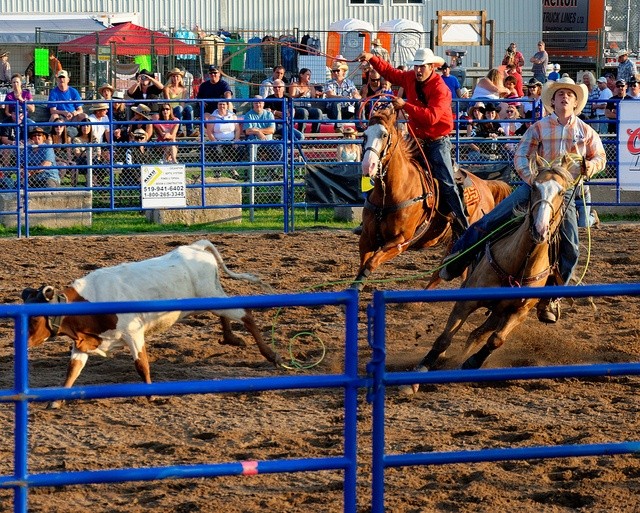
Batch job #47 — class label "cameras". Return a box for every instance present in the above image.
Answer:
[141,76,146,80]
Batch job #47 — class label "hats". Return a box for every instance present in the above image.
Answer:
[405,48,445,65]
[616,77,627,83]
[437,61,450,69]
[98,82,115,93]
[130,128,145,136]
[58,69,69,78]
[519,110,532,118]
[89,98,109,110]
[0,47,11,58]
[167,67,185,76]
[479,102,501,114]
[208,64,219,70]
[616,48,627,58]
[33,127,48,134]
[136,68,154,79]
[329,61,348,70]
[596,76,607,83]
[553,63,561,69]
[468,101,486,114]
[460,87,468,97]
[371,38,382,45]
[525,77,543,87]
[131,103,152,119]
[627,76,640,84]
[541,76,589,115]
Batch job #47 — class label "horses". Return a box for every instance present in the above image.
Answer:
[397,151,575,398]
[350,108,512,312]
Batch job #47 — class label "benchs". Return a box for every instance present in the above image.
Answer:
[450,129,468,157]
[149,135,282,158]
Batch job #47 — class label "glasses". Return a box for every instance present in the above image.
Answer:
[616,84,625,87]
[628,83,636,86]
[134,136,144,139]
[208,71,219,74]
[162,107,171,110]
[505,108,514,113]
[331,69,341,72]
[370,76,381,82]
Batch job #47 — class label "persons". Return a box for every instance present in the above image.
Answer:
[592,77,613,133]
[0,105,24,146]
[468,103,503,160]
[25,61,35,86]
[605,73,618,96]
[336,127,361,163]
[0,50,12,87]
[23,127,60,189]
[127,69,163,111]
[242,95,284,180]
[419,76,607,323]
[163,67,199,137]
[514,109,534,137]
[548,64,561,80]
[509,43,524,75]
[467,101,486,151]
[615,49,637,82]
[324,61,362,132]
[154,104,180,163]
[369,38,391,65]
[206,97,241,180]
[504,76,519,106]
[627,77,639,99]
[98,83,115,117]
[73,119,105,187]
[471,69,512,107]
[50,50,63,82]
[104,92,129,142]
[397,65,405,72]
[521,78,542,113]
[534,107,541,119]
[360,69,383,116]
[122,129,151,186]
[331,55,346,78]
[48,70,89,122]
[581,72,600,133]
[530,40,548,83]
[121,104,152,142]
[506,64,525,97]
[440,62,462,98]
[378,79,395,99]
[5,73,35,117]
[562,73,569,78]
[453,87,470,119]
[503,47,518,71]
[86,103,110,160]
[500,104,524,152]
[354,48,470,238]
[264,79,304,141]
[289,68,323,139]
[605,78,633,133]
[48,118,78,187]
[197,65,234,113]
[259,66,285,98]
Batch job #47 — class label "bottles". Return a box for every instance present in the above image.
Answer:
[126,147,133,165]
[490,137,497,160]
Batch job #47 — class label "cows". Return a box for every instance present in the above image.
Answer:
[20,239,283,409]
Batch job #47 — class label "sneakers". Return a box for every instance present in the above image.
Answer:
[441,254,457,281]
[590,208,601,229]
[537,281,558,322]
